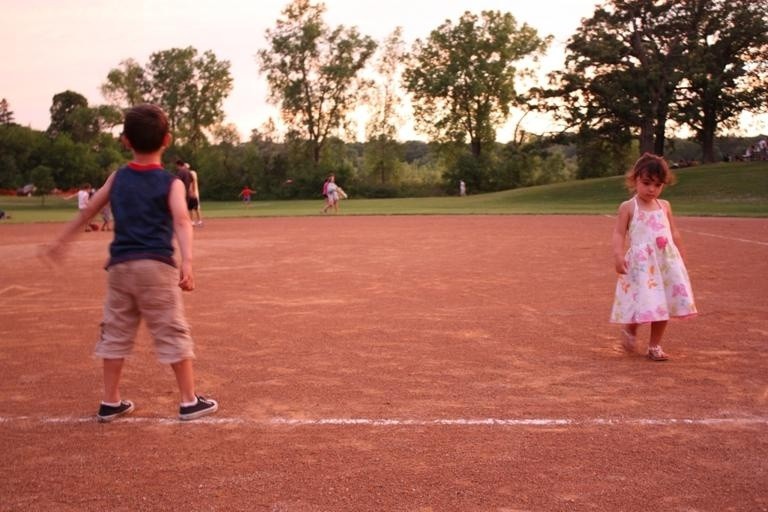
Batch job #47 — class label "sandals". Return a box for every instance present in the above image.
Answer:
[622,329,669,361]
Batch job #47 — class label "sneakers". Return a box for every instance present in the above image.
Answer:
[97,400,135,421]
[178,393,218,420]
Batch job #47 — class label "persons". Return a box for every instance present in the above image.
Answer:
[612,153,697,361]
[459,179,466,198]
[672,159,696,167]
[320,173,343,215]
[39,105,218,423]
[238,186,257,205]
[723,138,768,162]
[77,161,201,233]
